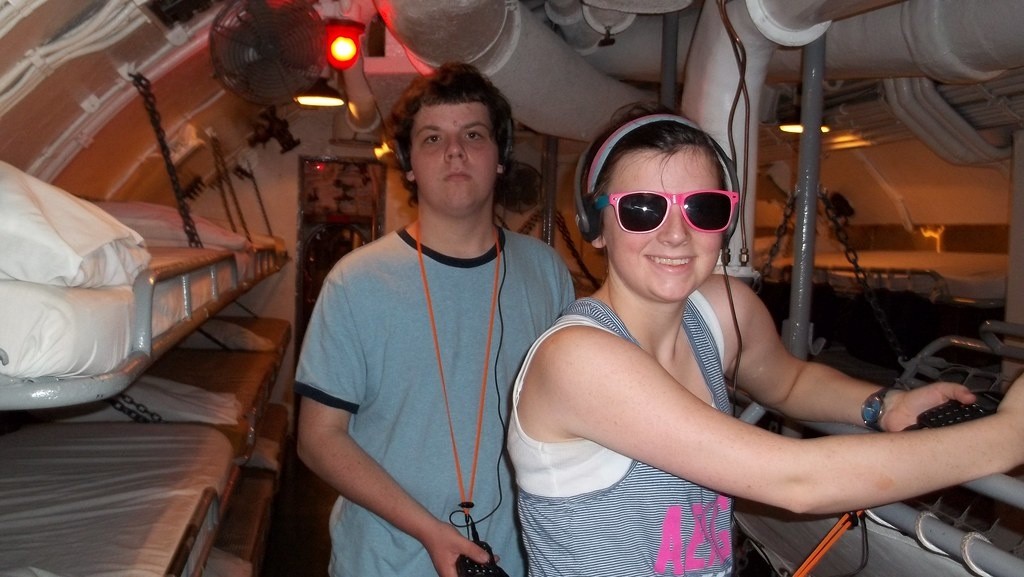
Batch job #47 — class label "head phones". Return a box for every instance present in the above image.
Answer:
[390,117,515,173]
[573,134,739,248]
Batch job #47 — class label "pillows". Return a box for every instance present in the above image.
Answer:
[83,199,246,250]
[243,434,282,472]
[182,325,273,350]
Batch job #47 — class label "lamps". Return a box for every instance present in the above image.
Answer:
[296,76,343,106]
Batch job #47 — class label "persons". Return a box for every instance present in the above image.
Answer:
[506,102,1024,577]
[291,62,575,577]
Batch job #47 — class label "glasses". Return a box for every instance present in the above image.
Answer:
[596,189,740,235]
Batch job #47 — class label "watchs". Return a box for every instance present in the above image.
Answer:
[861,386,892,432]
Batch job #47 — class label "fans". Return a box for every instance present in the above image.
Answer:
[204,0,328,109]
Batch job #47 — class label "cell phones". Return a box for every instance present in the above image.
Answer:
[916,392,1008,431]
[458,540,499,577]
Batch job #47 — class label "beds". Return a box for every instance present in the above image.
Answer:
[0,233,286,410]
[0,317,293,577]
[201,401,292,577]
